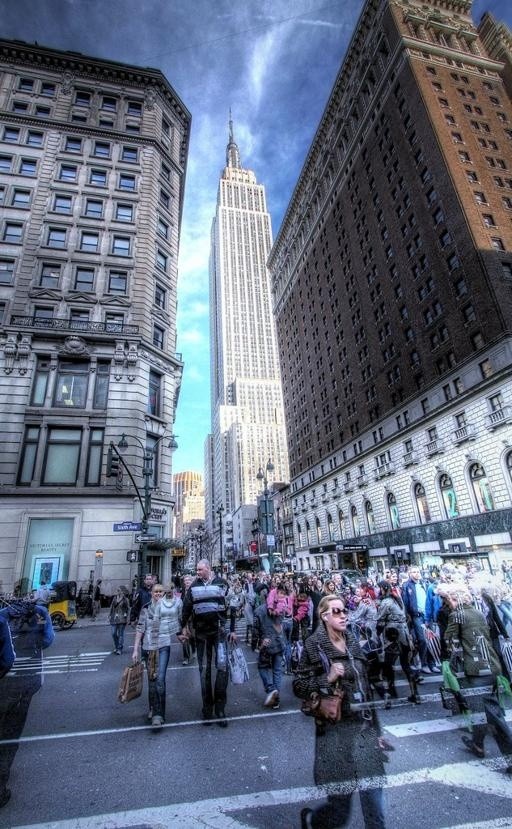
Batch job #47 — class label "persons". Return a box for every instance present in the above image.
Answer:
[0,560,512,829]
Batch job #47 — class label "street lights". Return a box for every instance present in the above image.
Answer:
[217,503,225,580]
[118,432,180,619]
[196,523,206,559]
[256,456,275,575]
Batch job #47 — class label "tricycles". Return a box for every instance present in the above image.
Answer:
[9,579,77,634]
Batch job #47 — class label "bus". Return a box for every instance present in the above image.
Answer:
[235,553,282,572]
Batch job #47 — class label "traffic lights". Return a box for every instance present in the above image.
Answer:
[106,451,118,477]
[128,549,141,561]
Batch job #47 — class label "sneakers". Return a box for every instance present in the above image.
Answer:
[152,716,163,732]
[262,689,282,710]
[408,695,421,704]
[462,736,484,758]
[148,710,152,718]
[432,667,441,672]
[113,647,121,655]
[422,666,432,674]
[182,653,196,665]
[393,665,422,671]
[385,693,392,709]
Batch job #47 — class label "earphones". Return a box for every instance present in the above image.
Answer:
[322,616,329,622]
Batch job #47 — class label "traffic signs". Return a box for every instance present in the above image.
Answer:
[114,521,142,531]
[135,534,156,542]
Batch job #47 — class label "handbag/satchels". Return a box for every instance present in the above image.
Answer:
[301,688,344,723]
[116,661,144,705]
[258,638,272,650]
[228,647,249,685]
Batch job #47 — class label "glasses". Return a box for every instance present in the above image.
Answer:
[322,607,349,615]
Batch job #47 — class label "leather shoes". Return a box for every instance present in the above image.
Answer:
[301,808,313,829]
[203,711,213,726]
[215,707,227,728]
